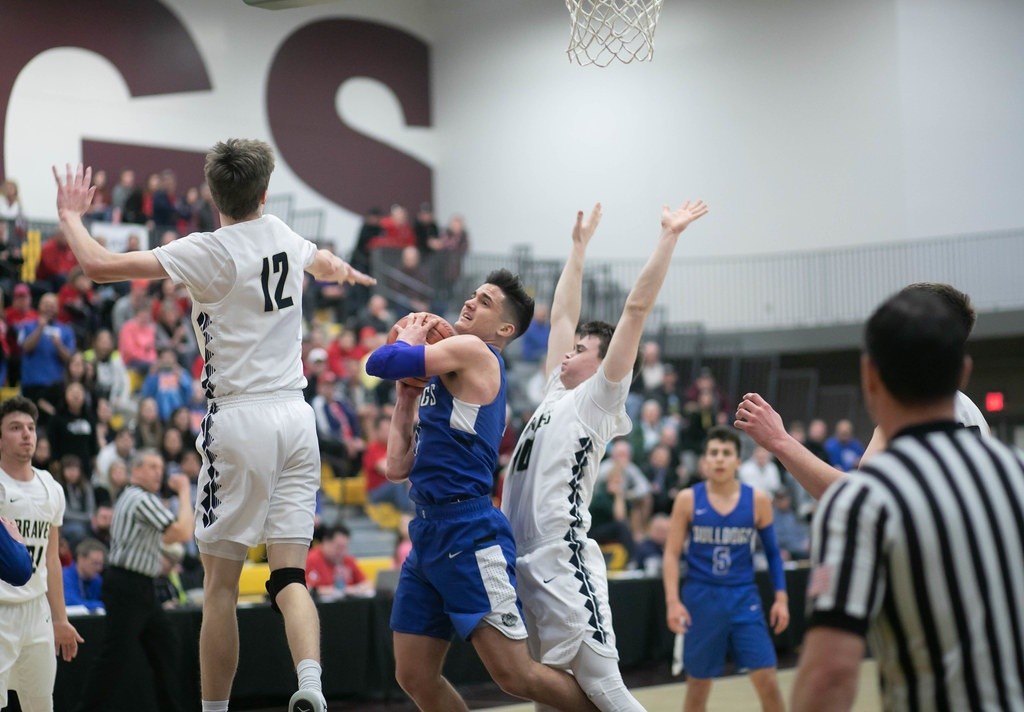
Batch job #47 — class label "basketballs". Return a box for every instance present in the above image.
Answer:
[385,310,458,387]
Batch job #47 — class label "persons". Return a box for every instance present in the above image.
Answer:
[662,428,789,712]
[501,200,710,712]
[366,267,599,712]
[51,139,375,712]
[733,284,1024,712]
[0,168,734,712]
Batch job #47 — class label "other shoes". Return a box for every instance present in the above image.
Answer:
[289,689,327,712]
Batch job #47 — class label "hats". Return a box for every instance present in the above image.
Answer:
[14,283,30,296]
[131,279,150,289]
[308,347,328,363]
[317,371,336,382]
